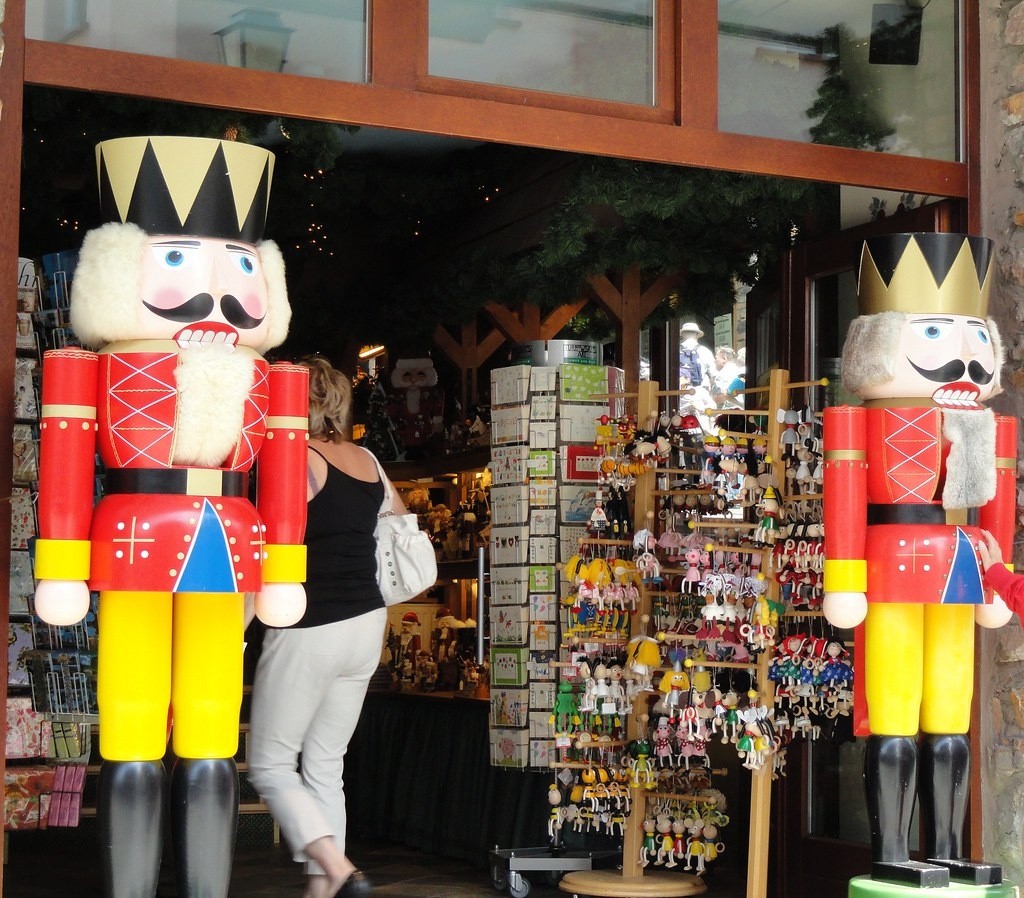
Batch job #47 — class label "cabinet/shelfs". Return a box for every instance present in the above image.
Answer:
[371,445,492,698]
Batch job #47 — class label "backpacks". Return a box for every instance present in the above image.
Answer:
[680,343,703,389]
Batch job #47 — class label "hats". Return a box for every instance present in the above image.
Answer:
[680,322,704,338]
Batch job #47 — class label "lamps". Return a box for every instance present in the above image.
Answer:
[208,7,300,75]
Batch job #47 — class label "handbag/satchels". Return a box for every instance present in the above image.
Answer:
[362,444,439,607]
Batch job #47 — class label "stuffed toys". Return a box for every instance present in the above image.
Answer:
[547,406,855,878]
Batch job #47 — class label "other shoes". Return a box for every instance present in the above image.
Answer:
[333,869,372,898]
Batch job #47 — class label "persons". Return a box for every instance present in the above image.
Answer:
[31,136,307,898]
[821,231,1018,884]
[244,355,413,898]
